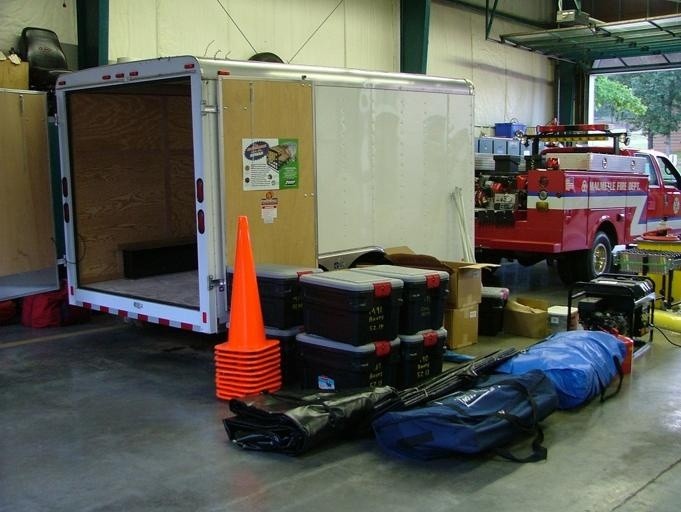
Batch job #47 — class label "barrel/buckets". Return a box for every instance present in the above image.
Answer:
[547,305,579,337]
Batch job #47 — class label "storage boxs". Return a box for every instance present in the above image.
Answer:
[344,264,451,337]
[385,247,501,308]
[225,321,301,388]
[295,331,401,391]
[548,306,579,331]
[301,269,403,347]
[474,123,544,171]
[445,304,479,349]
[504,297,548,337]
[397,328,448,389]
[226,260,323,331]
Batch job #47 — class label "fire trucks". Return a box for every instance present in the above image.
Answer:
[473,117,680,283]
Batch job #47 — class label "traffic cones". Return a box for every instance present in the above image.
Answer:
[213,214,283,404]
